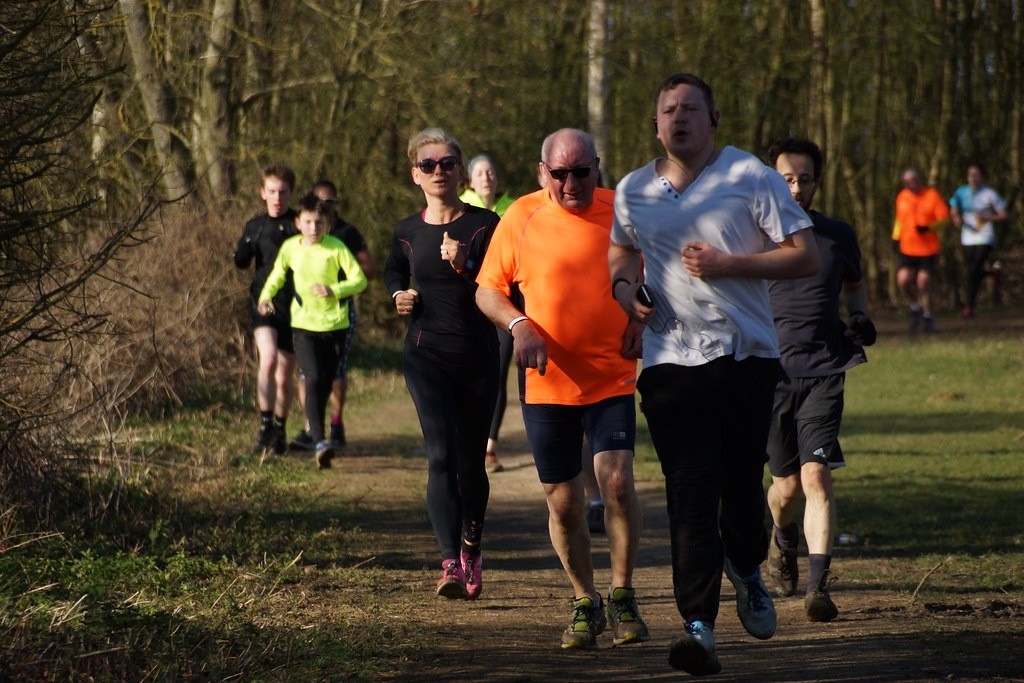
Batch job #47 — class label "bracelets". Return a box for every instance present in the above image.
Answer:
[611,278,631,301]
[508,316,528,334]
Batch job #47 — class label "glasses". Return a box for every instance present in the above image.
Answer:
[415,155,460,174]
[542,158,597,180]
[320,198,337,205]
[783,175,816,190]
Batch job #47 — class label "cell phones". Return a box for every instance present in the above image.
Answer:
[636,285,654,308]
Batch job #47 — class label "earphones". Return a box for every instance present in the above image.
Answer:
[710,112,718,129]
[654,119,659,134]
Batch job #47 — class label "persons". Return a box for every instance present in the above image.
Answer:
[888,168,951,332]
[383,126,502,601]
[457,149,517,473]
[949,163,1010,321]
[289,179,377,452]
[765,132,879,624]
[232,162,302,456]
[256,194,370,471]
[606,73,822,676]
[473,127,654,650]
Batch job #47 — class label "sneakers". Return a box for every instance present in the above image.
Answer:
[435,559,467,600]
[459,548,482,601]
[668,621,722,677]
[605,583,650,644]
[765,525,799,597]
[724,559,777,640]
[560,591,608,651]
[804,569,838,622]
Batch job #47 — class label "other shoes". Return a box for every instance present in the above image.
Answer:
[257,420,273,444]
[330,423,345,449]
[924,316,934,333]
[271,428,286,455]
[315,440,334,470]
[963,306,973,318]
[909,307,922,330]
[289,429,314,451]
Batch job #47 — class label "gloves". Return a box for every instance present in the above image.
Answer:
[845,309,878,347]
